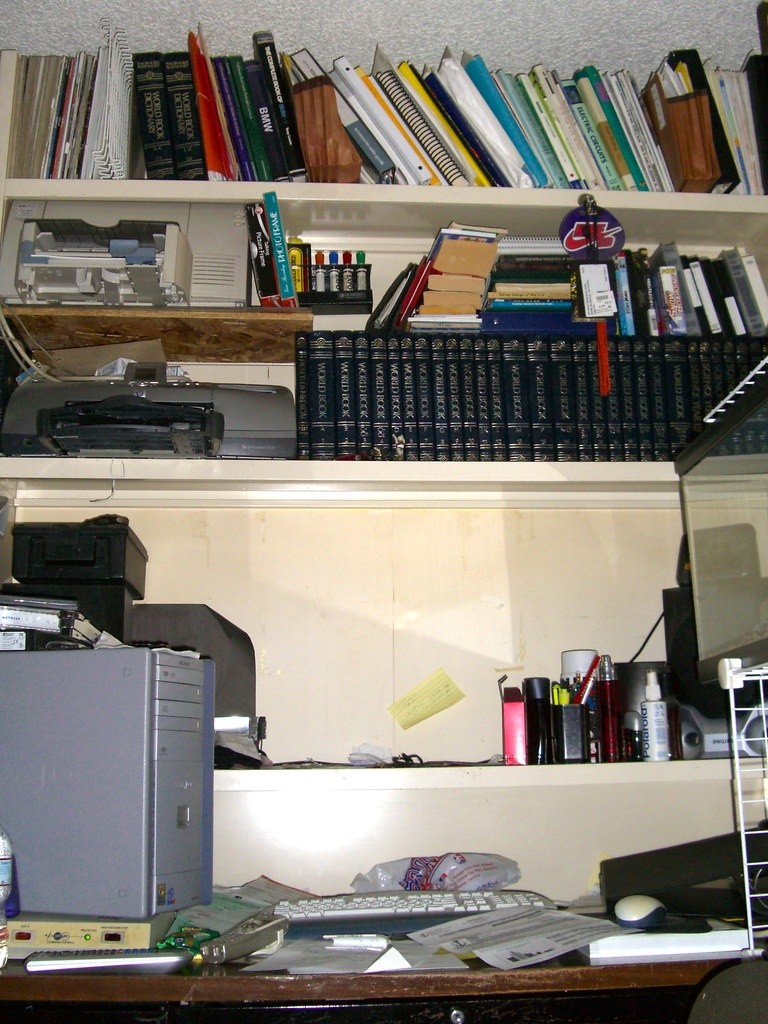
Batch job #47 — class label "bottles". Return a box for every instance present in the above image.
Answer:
[356,251,367,291]
[328,251,340,292]
[315,251,327,292]
[595,655,620,762]
[641,668,671,762]
[524,677,553,764]
[342,252,354,291]
[658,666,683,760]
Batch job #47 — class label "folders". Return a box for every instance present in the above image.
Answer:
[291,48,397,187]
[667,49,742,194]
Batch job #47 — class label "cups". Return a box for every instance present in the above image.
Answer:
[552,704,591,764]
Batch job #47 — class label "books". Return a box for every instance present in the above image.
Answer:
[2,22,768,195]
[576,921,749,957]
[369,221,768,337]
[293,330,767,462]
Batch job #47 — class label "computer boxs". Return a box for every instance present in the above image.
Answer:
[0,648,217,916]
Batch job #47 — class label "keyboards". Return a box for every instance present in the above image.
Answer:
[256,888,557,924]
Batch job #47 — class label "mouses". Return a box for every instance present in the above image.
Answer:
[614,895,668,928]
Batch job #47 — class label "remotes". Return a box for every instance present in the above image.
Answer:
[24,947,194,974]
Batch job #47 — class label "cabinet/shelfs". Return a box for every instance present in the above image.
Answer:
[0,173,766,796]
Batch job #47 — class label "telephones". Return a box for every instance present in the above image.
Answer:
[201,913,289,965]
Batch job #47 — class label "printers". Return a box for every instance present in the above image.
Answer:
[14,218,191,307]
[2,363,300,460]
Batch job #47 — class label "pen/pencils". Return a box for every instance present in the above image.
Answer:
[550,654,603,705]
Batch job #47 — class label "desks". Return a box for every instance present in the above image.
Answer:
[3,914,756,1005]
